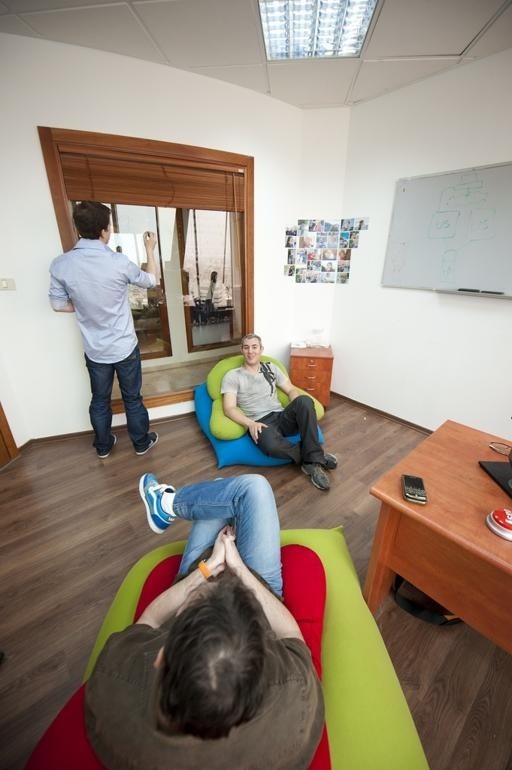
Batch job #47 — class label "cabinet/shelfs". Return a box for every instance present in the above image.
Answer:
[288,342,334,413]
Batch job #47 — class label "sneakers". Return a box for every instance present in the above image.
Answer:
[139,473,177,535]
[325,452,338,469]
[135,431,158,455]
[301,462,330,490]
[95,433,116,459]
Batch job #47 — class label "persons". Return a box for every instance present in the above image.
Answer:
[47,201,159,458]
[220,334,338,490]
[84,472,325,770]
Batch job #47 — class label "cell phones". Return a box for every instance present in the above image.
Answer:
[403,475,428,504]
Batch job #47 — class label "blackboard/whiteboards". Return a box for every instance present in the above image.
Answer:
[379,161,512,300]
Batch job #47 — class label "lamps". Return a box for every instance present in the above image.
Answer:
[256,0,382,62]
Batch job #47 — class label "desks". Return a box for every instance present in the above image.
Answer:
[358,417,511,660]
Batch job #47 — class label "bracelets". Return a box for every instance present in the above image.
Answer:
[197,558,214,582]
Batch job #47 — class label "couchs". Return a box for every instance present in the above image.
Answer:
[191,352,329,471]
[28,521,435,770]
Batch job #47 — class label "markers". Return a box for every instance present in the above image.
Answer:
[458,288,504,295]
